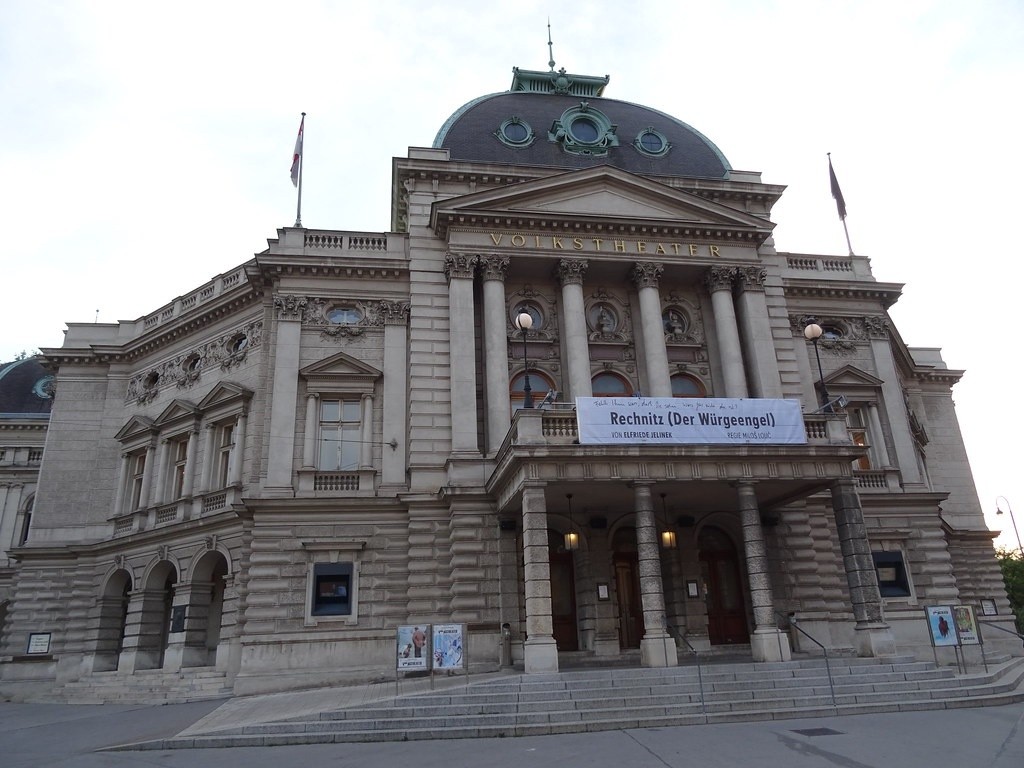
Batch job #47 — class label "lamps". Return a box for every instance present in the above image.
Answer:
[563,493,581,552]
[659,493,678,550]
[538,389,559,408]
[804,319,834,414]
[676,514,696,529]
[759,515,779,528]
[499,518,517,531]
[810,393,850,414]
[514,306,539,408]
[590,517,607,529]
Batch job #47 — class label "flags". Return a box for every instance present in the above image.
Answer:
[829,159,847,221]
[290,118,303,187]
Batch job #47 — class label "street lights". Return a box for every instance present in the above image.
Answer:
[996,495,1023,556]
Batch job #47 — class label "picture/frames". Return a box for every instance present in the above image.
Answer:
[686,579,699,599]
[596,582,611,602]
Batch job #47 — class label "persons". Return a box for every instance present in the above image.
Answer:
[403,627,427,658]
[599,310,611,325]
[669,314,681,328]
